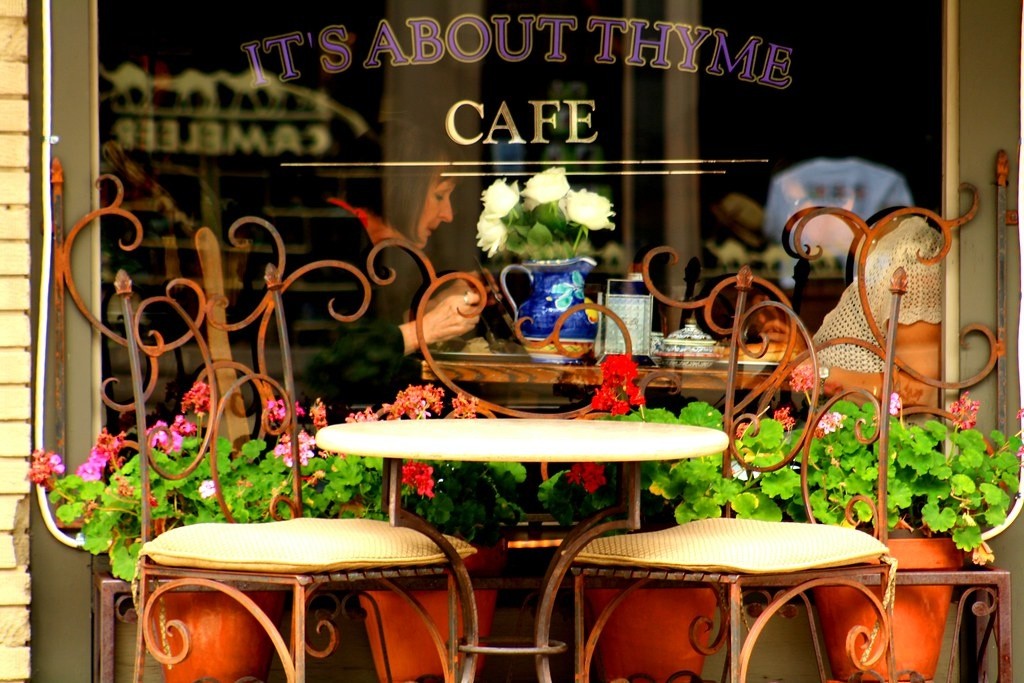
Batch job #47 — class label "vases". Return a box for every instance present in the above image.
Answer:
[813,531,963,683]
[585,588,717,683]
[359,540,507,683]
[500,255,599,364]
[150,579,287,683]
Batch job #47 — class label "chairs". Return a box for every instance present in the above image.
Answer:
[114,263,477,683]
[563,264,907,683]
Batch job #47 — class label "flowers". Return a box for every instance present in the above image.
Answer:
[27,383,526,582]
[476,166,616,261]
[538,354,1024,566]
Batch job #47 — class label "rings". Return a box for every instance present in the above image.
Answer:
[464,291,469,304]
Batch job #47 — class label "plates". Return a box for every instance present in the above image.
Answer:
[651,357,715,369]
[716,360,780,372]
[423,349,532,363]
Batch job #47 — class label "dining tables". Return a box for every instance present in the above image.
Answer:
[410,357,791,392]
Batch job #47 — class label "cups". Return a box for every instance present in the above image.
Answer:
[663,323,715,353]
[650,332,663,355]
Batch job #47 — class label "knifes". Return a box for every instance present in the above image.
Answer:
[470,255,517,338]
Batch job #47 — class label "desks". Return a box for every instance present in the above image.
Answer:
[315,419,729,683]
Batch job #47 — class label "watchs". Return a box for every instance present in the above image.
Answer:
[818,362,832,396]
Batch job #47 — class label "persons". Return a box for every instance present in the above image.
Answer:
[759,211,940,428]
[253,118,502,425]
